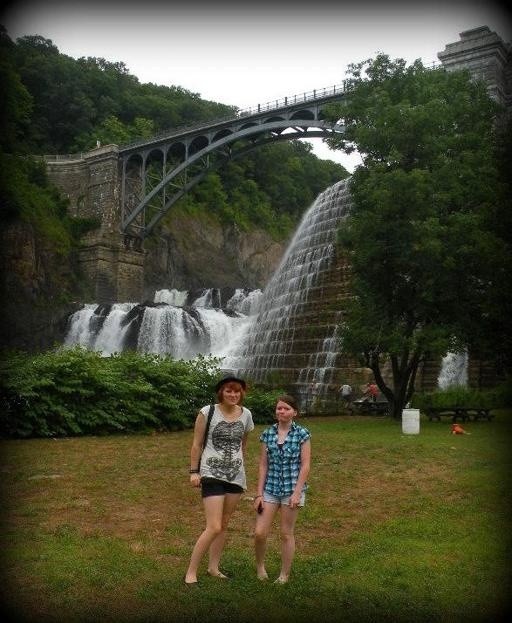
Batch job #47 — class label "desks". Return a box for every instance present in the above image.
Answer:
[353,401,389,415]
[424,407,494,423]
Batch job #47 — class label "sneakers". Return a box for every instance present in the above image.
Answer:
[273,577,287,585]
[256,573,268,581]
[183,576,201,589]
[207,569,227,578]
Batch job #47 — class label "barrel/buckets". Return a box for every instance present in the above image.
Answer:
[401,408,420,435]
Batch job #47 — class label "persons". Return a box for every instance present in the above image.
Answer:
[368,382,380,401]
[338,382,353,399]
[359,382,372,402]
[253,393,312,586]
[183,372,256,588]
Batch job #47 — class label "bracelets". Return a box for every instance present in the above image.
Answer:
[189,469,199,473]
[255,494,262,498]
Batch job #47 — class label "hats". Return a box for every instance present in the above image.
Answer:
[215,376,246,392]
